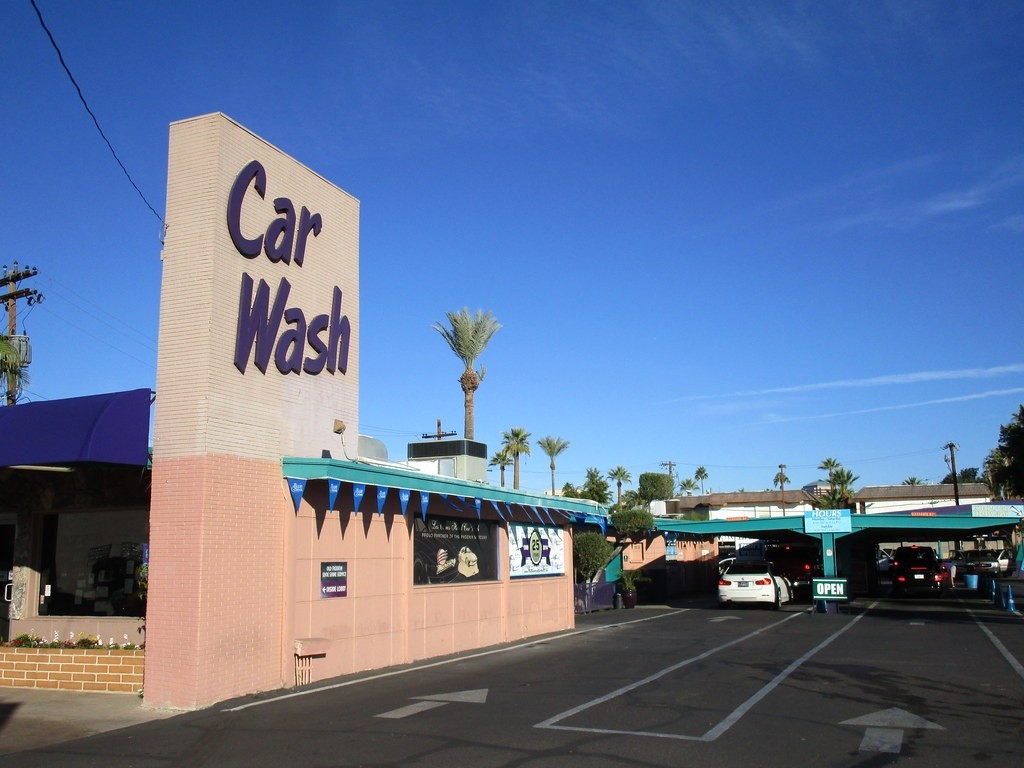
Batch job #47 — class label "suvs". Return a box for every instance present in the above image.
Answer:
[888,544,945,598]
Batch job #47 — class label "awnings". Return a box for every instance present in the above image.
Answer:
[282,457,610,525]
[1,386,152,472]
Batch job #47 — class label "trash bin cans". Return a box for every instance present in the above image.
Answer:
[964,575,978,588]
[613,592,623,609]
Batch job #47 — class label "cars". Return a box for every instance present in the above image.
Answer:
[718,563,795,609]
[949,549,1010,580]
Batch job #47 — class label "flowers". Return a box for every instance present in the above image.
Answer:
[0,628,145,650]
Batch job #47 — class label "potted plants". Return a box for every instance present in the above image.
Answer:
[616,569,652,609]
